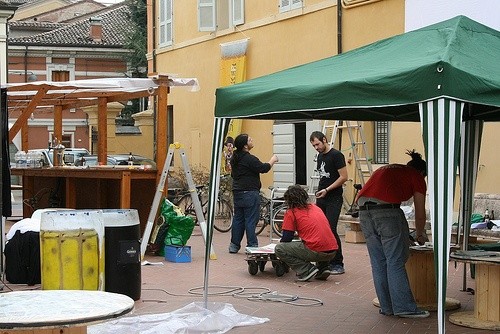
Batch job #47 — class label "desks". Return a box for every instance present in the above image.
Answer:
[0,291,134,334]
[9,164,167,224]
[374,246,462,313]
[449,252,500,331]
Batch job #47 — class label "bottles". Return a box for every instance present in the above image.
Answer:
[81,155,85,166]
[127,152,134,166]
[483,208,489,223]
[489,210,494,220]
[15,150,49,167]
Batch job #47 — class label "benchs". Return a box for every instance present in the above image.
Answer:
[453,193,500,239]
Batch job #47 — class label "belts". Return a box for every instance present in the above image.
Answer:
[359,203,400,211]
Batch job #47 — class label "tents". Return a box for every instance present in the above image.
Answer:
[203,14,500,334]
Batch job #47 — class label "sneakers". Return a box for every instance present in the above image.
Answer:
[331,262,344,274]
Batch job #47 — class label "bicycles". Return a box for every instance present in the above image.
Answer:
[254,185,299,240]
[176,173,234,232]
[342,178,362,218]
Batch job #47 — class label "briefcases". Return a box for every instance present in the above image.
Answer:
[164,237,192,263]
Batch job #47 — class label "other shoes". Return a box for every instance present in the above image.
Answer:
[315,265,331,280]
[399,310,430,318]
[297,263,320,281]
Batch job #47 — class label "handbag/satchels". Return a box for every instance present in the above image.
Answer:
[164,215,196,246]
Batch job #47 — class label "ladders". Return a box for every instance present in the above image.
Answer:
[309,119,374,204]
[140,141,216,260]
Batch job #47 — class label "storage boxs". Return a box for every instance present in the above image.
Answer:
[344,223,366,243]
[165,237,191,263]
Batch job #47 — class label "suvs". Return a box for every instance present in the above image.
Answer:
[40,148,92,166]
[74,154,153,165]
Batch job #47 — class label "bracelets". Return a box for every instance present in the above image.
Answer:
[325,188,328,193]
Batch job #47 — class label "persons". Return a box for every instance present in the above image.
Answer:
[355,149,429,318]
[229,133,279,254]
[310,131,349,275]
[275,184,338,282]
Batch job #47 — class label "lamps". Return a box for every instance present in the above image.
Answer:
[20,70,39,82]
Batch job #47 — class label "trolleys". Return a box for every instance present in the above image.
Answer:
[244,188,308,277]
